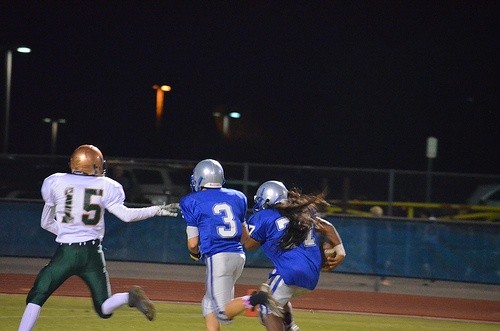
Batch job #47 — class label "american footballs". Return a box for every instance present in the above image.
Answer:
[318,236,336,271]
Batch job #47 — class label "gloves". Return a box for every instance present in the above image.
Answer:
[190,251,202,261]
[155,201,181,217]
[327,244,347,270]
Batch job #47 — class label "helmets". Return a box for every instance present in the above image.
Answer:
[70,144,106,176]
[254,180,288,207]
[190,159,225,192]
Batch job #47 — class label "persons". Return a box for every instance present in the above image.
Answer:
[245,180,347,330]
[19,144,179,331]
[180,158,285,331]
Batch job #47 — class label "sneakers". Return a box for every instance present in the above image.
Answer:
[249,282,284,319]
[129,285,155,321]
